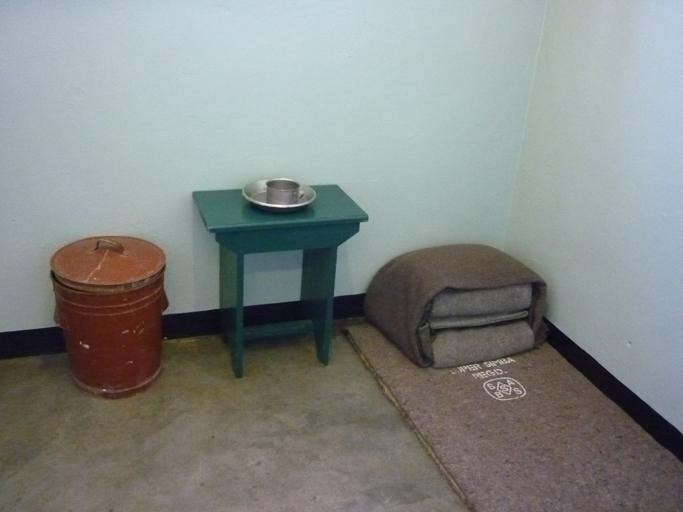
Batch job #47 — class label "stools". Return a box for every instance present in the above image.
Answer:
[190,182,370,377]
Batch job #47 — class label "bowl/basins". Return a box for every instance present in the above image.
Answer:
[241,178,317,211]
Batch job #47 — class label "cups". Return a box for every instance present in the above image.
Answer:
[265,180,300,205]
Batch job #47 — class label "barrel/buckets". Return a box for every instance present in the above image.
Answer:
[49,236,170,403]
